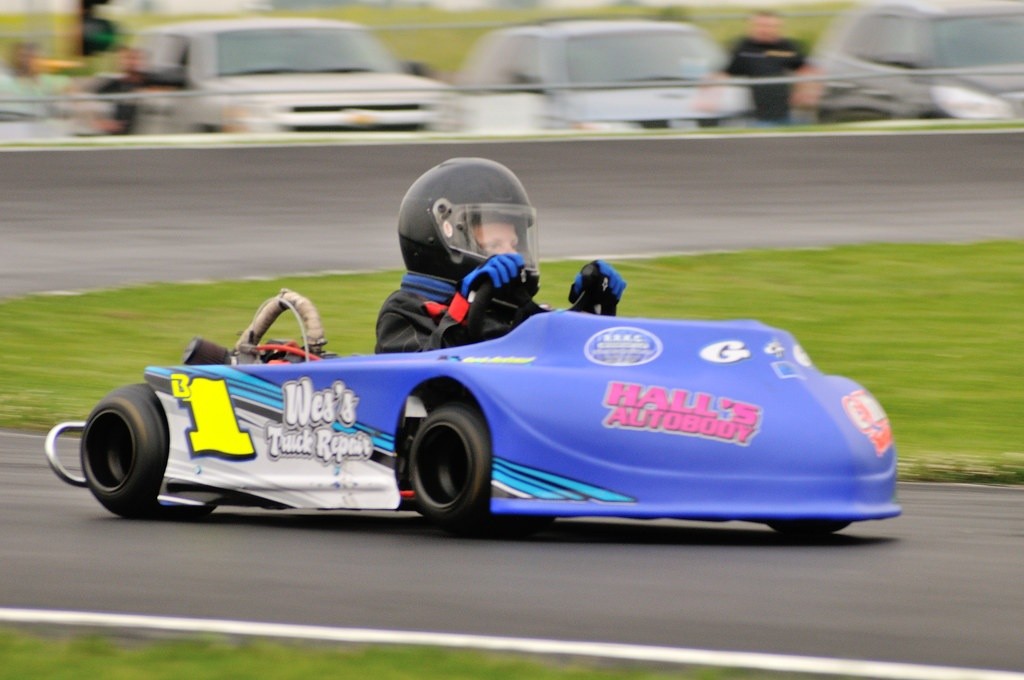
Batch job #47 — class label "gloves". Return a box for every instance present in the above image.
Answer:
[568,258,628,314]
[456,252,527,303]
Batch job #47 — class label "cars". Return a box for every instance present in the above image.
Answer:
[464,20,756,130]
[137,19,458,132]
[787,1,1024,124]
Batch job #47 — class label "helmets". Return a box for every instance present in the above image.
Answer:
[396,157,541,304]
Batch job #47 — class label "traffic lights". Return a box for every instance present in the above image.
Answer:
[79,0,115,62]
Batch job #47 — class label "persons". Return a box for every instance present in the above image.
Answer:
[694,10,822,123]
[375,157,628,354]
